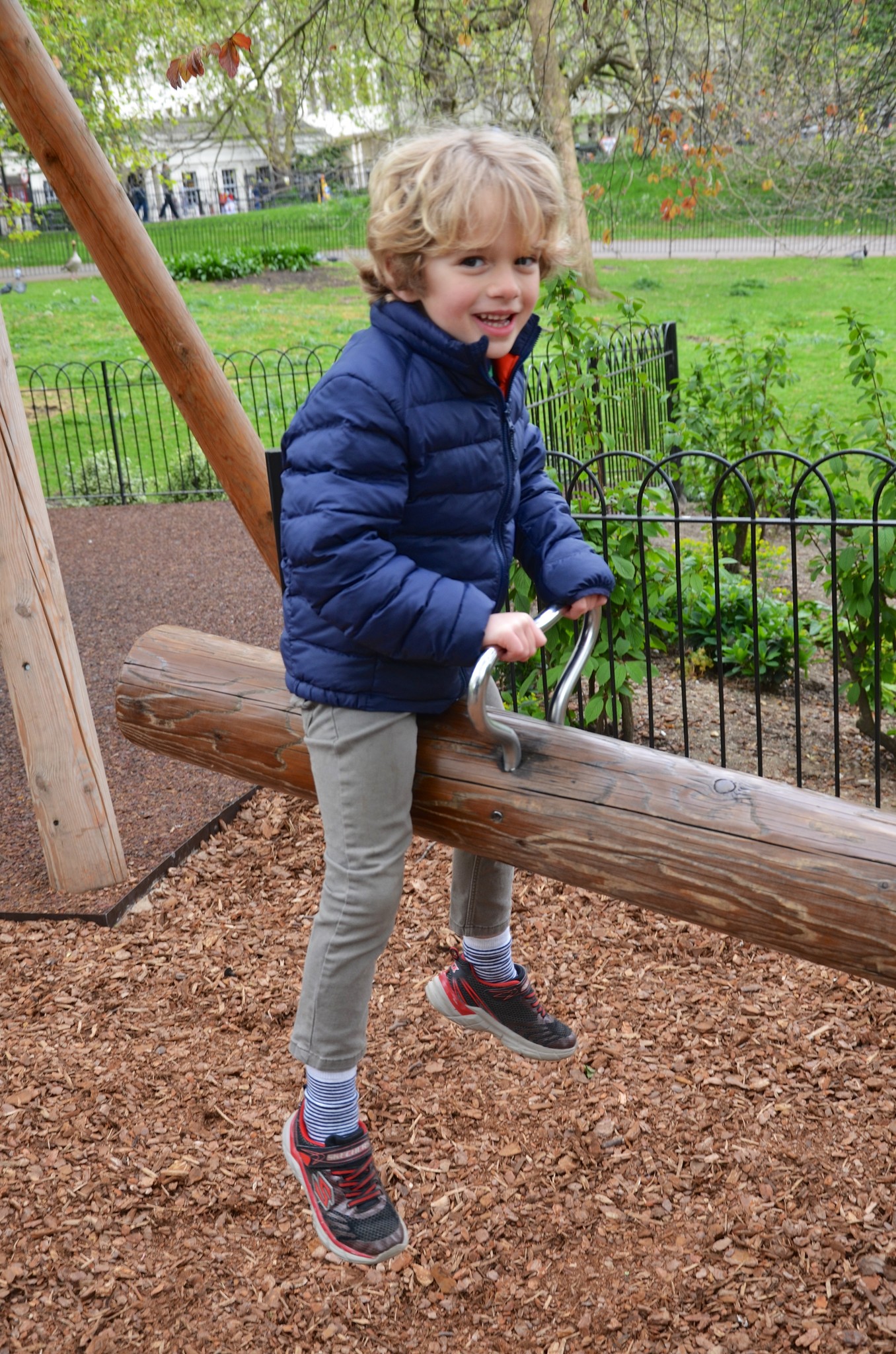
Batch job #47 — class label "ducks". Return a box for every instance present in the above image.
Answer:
[59,238,84,280]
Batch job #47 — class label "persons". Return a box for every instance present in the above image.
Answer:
[125,164,273,221]
[278,127,617,1266]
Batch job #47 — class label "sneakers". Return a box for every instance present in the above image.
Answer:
[281,1098,407,1265]
[424,947,577,1060]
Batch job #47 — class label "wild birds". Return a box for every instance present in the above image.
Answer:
[0,266,29,295]
